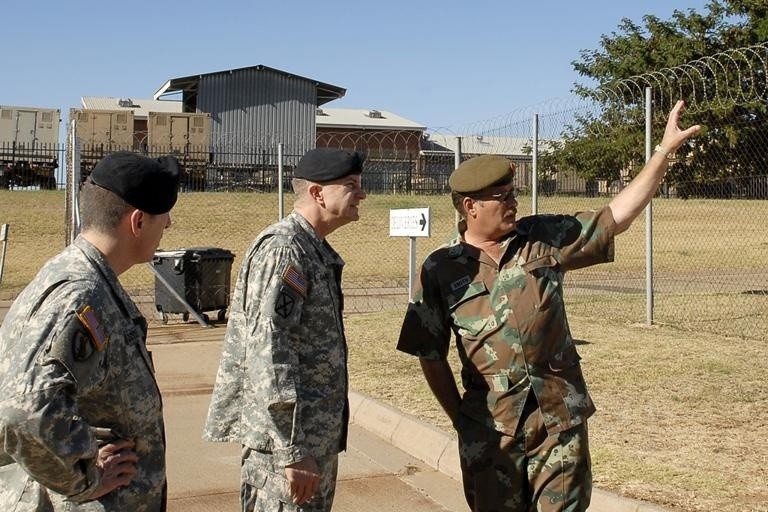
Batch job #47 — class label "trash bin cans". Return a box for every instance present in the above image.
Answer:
[154,248,236,324]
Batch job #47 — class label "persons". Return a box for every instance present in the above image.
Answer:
[0,152,186,512]
[202,147,366,512]
[398,99,701,512]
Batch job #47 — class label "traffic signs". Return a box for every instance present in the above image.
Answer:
[387,207,431,237]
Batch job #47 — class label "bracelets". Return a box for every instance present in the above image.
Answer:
[654,145,670,159]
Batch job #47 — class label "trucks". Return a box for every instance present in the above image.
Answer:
[147,109,210,191]
[0,103,58,189]
[68,108,133,185]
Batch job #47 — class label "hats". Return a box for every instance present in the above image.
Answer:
[448,155,515,196]
[294,149,365,182]
[90,151,179,215]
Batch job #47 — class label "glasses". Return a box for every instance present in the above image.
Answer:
[471,189,518,201]
